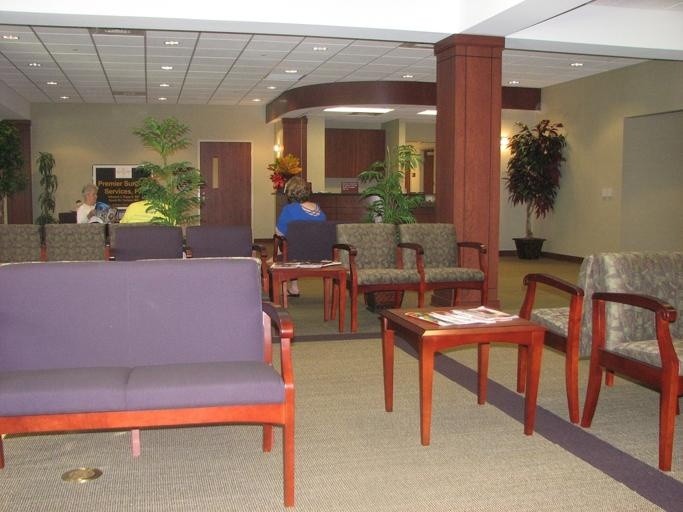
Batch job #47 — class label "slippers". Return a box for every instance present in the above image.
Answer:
[281,289,299,297]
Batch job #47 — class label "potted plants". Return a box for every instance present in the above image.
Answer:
[501,118,567,260]
[357,144,425,314]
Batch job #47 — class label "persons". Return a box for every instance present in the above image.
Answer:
[73,183,98,224]
[117,184,175,225]
[267,174,329,298]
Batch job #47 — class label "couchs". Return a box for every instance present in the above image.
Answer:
[0,257,296,509]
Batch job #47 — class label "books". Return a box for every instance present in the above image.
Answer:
[403,302,520,326]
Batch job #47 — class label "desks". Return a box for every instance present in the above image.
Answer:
[270,261,347,336]
[378,304,548,445]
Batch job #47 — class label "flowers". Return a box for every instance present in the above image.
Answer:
[267,153,302,189]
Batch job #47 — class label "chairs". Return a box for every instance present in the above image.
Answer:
[58,209,77,223]
[399,223,488,309]
[581,250,683,472]
[108,225,185,260]
[108,221,158,242]
[516,251,615,423]
[43,223,109,262]
[273,220,337,309]
[184,224,270,302]
[0,224,44,264]
[331,222,425,335]
